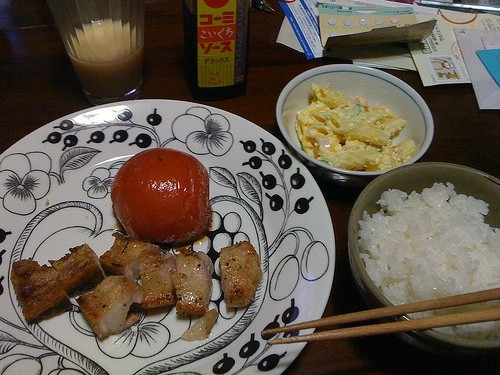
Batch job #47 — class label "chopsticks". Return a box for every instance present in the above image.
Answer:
[262,287,500,344]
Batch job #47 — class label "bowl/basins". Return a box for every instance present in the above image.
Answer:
[347,161,500,348]
[275,64,434,177]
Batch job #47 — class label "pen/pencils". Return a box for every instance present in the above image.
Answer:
[416,0,500,16]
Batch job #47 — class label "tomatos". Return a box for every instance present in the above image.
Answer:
[109,147,213,244]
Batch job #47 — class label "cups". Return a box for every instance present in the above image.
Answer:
[48,0,143,107]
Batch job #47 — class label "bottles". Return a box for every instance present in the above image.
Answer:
[184,0,252,101]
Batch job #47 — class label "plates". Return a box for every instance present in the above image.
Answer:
[0,98,335,375]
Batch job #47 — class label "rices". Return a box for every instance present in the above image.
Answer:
[357,182,500,339]
